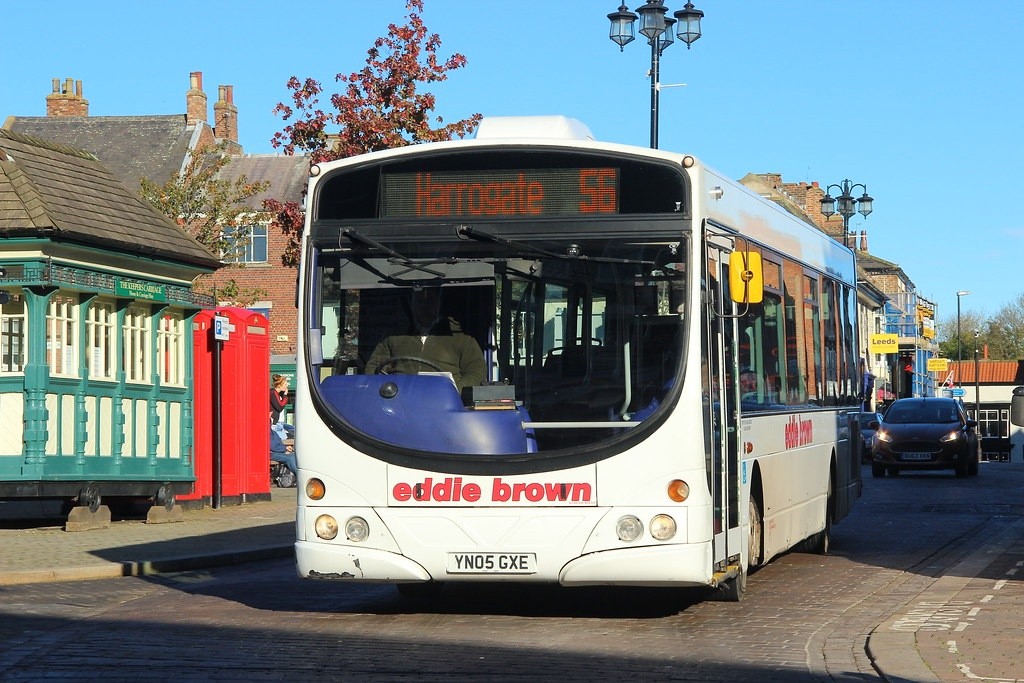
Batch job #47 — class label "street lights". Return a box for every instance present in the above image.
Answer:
[818,178,874,249]
[956,290,972,406]
[606,0,706,148]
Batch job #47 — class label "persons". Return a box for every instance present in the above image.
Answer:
[739,331,778,389]
[364,288,489,400]
[269,421,298,476]
[269,374,288,425]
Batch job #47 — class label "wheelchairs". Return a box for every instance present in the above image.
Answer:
[270,453,298,489]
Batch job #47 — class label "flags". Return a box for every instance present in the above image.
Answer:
[947,372,954,388]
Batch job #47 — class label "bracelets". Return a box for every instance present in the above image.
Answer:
[283,395,287,396]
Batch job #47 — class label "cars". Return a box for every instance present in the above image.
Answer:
[871,396,980,477]
[861,411,885,459]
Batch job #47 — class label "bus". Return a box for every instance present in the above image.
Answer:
[291,115,867,604]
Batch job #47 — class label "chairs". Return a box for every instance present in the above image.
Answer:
[526,311,853,434]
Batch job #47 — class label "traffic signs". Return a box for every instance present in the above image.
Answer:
[951,388,965,397]
